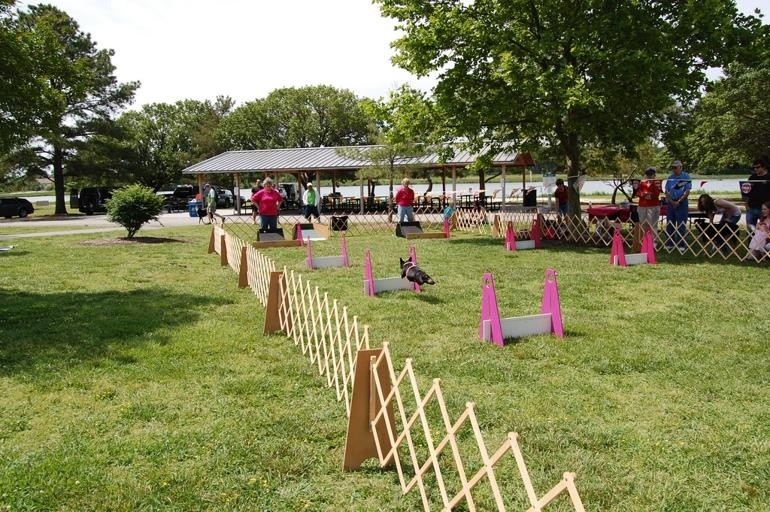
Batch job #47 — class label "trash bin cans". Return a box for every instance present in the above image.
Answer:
[521,189,537,207]
[188,201,203,217]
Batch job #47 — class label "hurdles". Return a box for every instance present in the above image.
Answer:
[296,223,326,247]
[609,229,655,267]
[306,233,349,269]
[477,269,565,348]
[504,221,541,251]
[363,248,422,297]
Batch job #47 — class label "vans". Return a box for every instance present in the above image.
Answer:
[277,182,309,202]
[69,184,127,216]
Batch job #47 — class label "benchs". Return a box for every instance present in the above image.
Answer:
[241,195,502,214]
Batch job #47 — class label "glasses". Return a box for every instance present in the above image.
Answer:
[647,173,654,175]
[753,166,761,169]
[671,166,677,169]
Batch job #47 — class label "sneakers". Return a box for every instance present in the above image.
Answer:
[653,242,686,252]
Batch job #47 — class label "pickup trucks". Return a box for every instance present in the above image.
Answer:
[155,183,244,212]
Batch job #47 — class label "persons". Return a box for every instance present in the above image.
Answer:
[395,178,414,223]
[663,160,692,251]
[303,183,321,224]
[555,179,570,226]
[748,203,770,261]
[747,160,770,243]
[252,179,264,225]
[698,194,742,251]
[204,183,225,225]
[251,177,285,229]
[636,168,661,248]
[750,222,767,261]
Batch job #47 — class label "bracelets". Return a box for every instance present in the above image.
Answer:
[678,198,684,204]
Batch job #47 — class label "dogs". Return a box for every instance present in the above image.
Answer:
[194,205,216,224]
[400,256,435,286]
[695,219,739,256]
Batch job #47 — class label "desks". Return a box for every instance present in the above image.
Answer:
[688,211,715,229]
[586,203,668,228]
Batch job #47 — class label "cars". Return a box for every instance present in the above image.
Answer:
[0,193,33,219]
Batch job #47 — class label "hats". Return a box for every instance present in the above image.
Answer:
[671,160,681,166]
[645,167,656,173]
[306,181,313,187]
